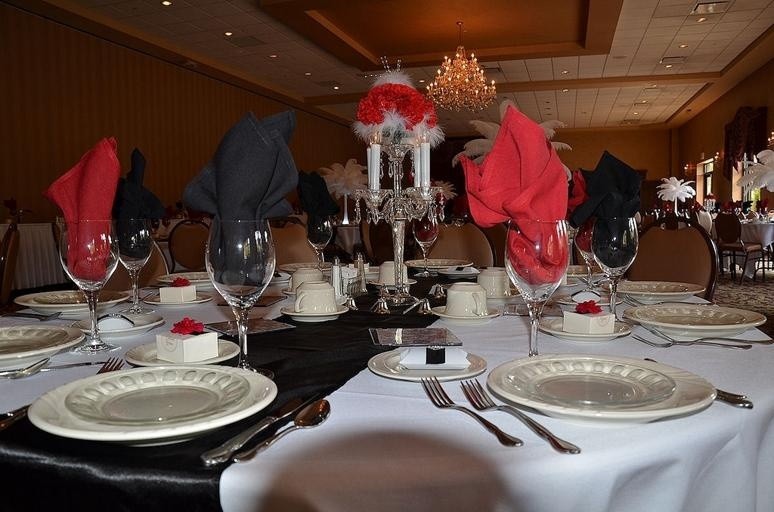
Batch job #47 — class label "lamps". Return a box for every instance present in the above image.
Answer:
[424,21,498,116]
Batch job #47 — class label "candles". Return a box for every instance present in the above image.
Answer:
[421,142,431,187]
[368,143,380,194]
[413,146,421,189]
[365,146,371,189]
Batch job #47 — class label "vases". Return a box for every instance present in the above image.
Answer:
[340,193,349,226]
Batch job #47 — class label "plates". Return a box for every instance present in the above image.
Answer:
[279,305,350,323]
[124,338,242,366]
[509,287,523,299]
[0,323,87,375]
[14,287,130,311]
[144,291,214,308]
[78,312,166,340]
[487,353,717,425]
[431,306,501,325]
[553,295,624,306]
[566,265,609,280]
[621,303,767,336]
[368,346,487,384]
[26,367,278,449]
[160,270,216,289]
[614,281,706,305]
[536,316,633,342]
[404,258,473,272]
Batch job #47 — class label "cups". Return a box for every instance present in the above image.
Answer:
[289,269,323,293]
[377,261,409,283]
[294,282,338,313]
[445,282,488,316]
[480,267,512,298]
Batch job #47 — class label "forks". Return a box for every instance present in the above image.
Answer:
[1,311,63,321]
[619,324,772,355]
[420,375,525,448]
[460,377,581,457]
[0,357,123,434]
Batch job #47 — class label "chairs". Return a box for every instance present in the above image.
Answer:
[370,200,774,301]
[0,207,373,293]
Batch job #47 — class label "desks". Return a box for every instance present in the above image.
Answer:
[0,276,773,511]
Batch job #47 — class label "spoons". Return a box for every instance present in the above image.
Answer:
[0,356,50,380]
[233,399,332,463]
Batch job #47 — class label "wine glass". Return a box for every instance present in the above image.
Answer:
[204,217,277,382]
[642,206,774,224]
[115,216,155,318]
[306,217,333,271]
[504,213,639,358]
[59,219,122,354]
[411,216,439,278]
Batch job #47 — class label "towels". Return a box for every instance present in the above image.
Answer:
[293,165,340,242]
[406,169,447,242]
[38,136,128,285]
[566,148,650,267]
[108,147,166,260]
[192,108,303,288]
[455,104,573,290]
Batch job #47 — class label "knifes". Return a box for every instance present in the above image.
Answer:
[57,317,83,322]
[643,357,754,409]
[199,391,324,466]
